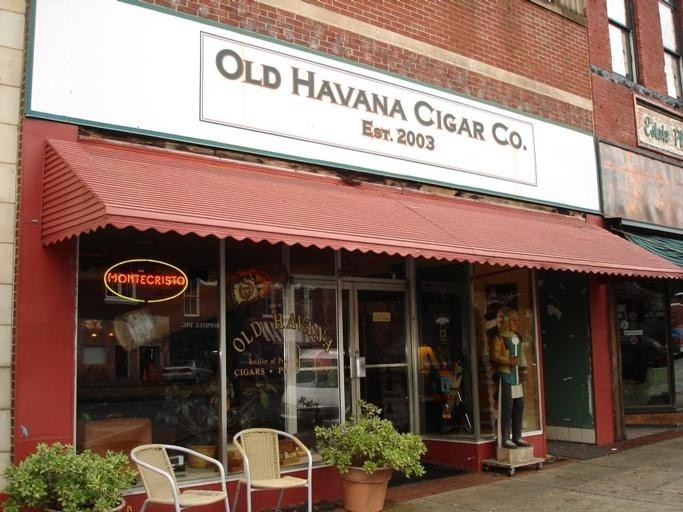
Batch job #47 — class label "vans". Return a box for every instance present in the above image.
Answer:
[280,348,352,424]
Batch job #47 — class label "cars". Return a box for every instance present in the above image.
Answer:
[620,335,683,380]
[162,365,213,385]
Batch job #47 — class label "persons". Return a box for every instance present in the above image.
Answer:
[490,305,533,447]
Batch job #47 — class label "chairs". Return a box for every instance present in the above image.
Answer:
[129,427,312,511]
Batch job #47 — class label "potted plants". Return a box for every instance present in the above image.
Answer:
[313,400,428,510]
[1,443,142,512]
